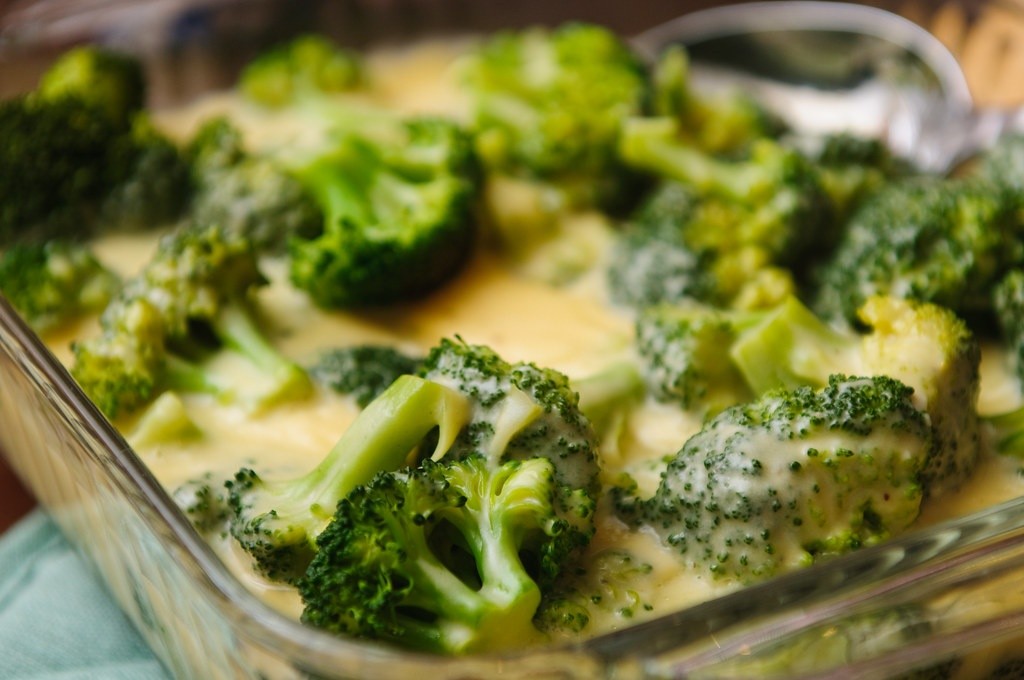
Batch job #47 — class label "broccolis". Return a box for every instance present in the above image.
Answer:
[0,18,1024,680]
